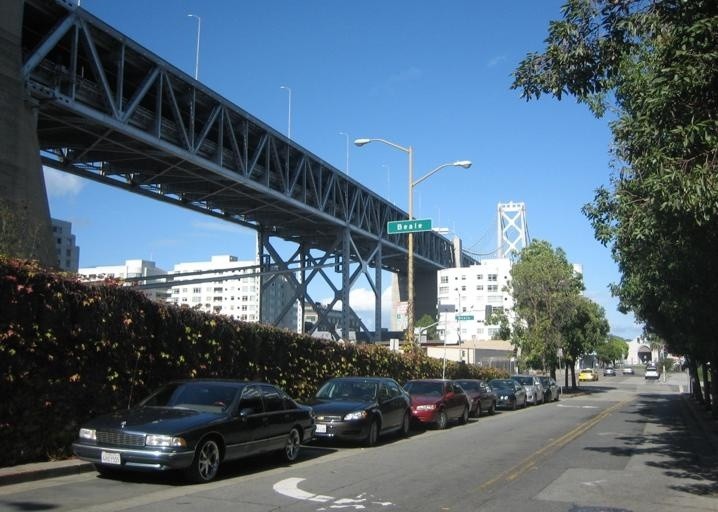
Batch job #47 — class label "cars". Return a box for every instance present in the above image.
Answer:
[452,379,496,418]
[71,378,316,484]
[487,375,559,410]
[643,368,658,380]
[603,367,615,376]
[578,368,597,382]
[300,376,411,447]
[402,378,469,430]
[622,367,633,375]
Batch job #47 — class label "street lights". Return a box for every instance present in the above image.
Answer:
[280,86,290,139]
[454,287,461,363]
[187,14,200,81]
[338,131,348,175]
[353,136,472,356]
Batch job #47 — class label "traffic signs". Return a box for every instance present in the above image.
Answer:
[386,218,431,235]
[455,315,473,320]
